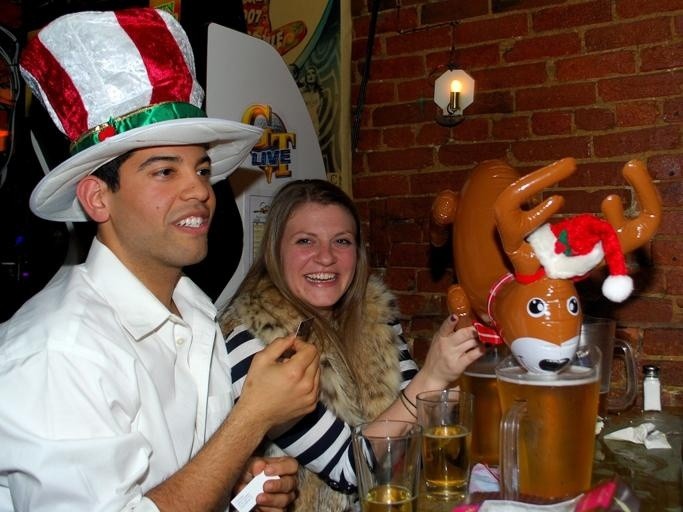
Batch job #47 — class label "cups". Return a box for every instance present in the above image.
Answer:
[579,316,637,417]
[495,346,603,506]
[352,421,423,512]
[416,389,474,512]
[462,360,509,467]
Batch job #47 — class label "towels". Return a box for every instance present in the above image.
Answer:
[600,418,673,452]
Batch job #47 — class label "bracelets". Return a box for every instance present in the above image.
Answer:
[401,389,416,409]
[400,395,417,420]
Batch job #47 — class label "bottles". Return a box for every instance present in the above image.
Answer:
[643,364,665,415]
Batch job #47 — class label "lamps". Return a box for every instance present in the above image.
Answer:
[427,24,478,128]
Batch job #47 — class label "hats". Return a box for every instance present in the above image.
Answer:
[17,4,266,225]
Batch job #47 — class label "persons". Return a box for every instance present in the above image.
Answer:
[217,178,493,512]
[0,146,323,512]
